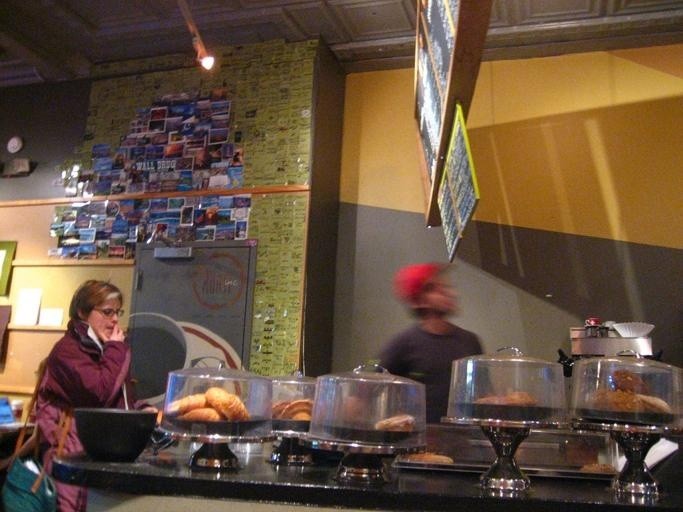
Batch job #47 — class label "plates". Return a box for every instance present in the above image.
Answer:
[454,400,561,422]
[317,424,422,444]
[272,417,310,432]
[169,417,268,435]
[576,408,680,427]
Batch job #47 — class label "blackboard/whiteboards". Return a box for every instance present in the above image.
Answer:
[412,0,493,262]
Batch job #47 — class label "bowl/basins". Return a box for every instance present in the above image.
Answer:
[73,407,166,462]
[613,322,656,338]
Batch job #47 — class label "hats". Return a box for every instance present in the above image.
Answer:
[391,262,451,301]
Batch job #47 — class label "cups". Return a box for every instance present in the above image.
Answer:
[585,318,601,327]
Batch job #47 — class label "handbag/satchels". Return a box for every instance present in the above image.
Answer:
[0,455,56,512]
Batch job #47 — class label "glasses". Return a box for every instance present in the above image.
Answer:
[94,307,124,317]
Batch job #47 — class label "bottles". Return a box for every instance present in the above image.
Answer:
[11,400,24,423]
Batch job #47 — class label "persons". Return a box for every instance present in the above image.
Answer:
[30,277,163,512]
[365,262,496,425]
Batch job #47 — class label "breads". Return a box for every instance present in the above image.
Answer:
[168,387,249,423]
[588,369,671,415]
[409,454,453,465]
[376,414,416,433]
[272,398,313,421]
[476,391,537,407]
[581,464,611,473]
